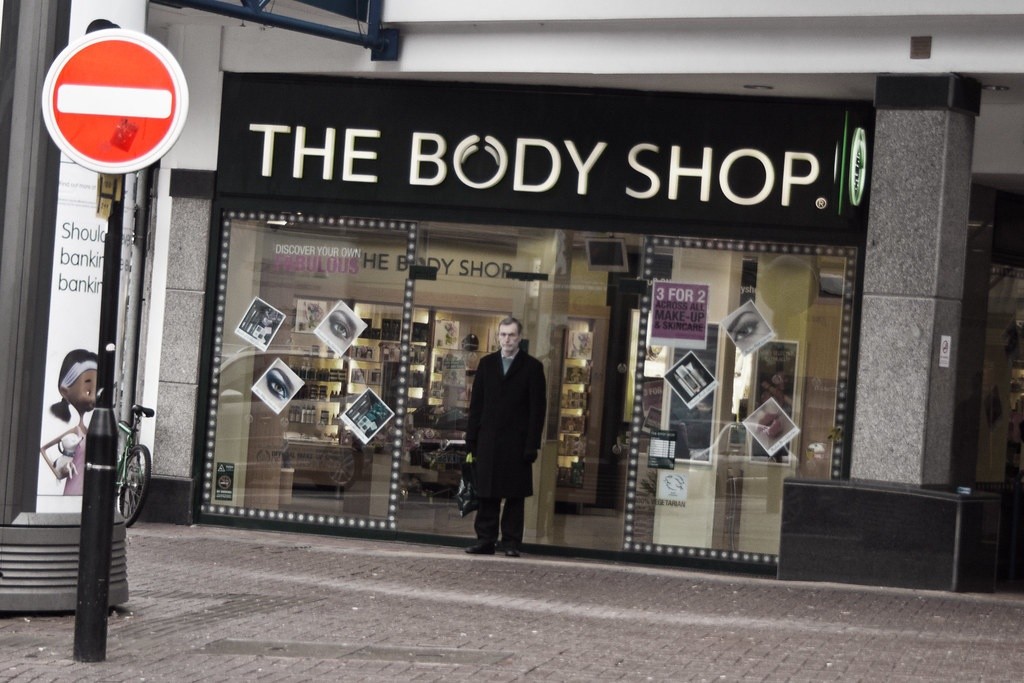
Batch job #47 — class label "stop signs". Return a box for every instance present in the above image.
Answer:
[43,29,189,176]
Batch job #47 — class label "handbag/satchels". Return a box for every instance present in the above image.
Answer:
[456,452,479,517]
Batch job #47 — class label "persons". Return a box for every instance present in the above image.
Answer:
[466,318,547,556]
[534,324,588,548]
[421,388,468,472]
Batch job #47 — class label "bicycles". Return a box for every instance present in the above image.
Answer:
[116,403,155,527]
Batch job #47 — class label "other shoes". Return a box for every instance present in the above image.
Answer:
[466,544,495,555]
[505,548,519,556]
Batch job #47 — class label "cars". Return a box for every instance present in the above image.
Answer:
[619,421,801,502]
[377,404,473,493]
[1009,321,1024,419]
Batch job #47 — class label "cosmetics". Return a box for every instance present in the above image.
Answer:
[675,362,706,397]
[284,345,372,424]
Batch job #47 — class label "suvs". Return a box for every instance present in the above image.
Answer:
[223,343,369,491]
